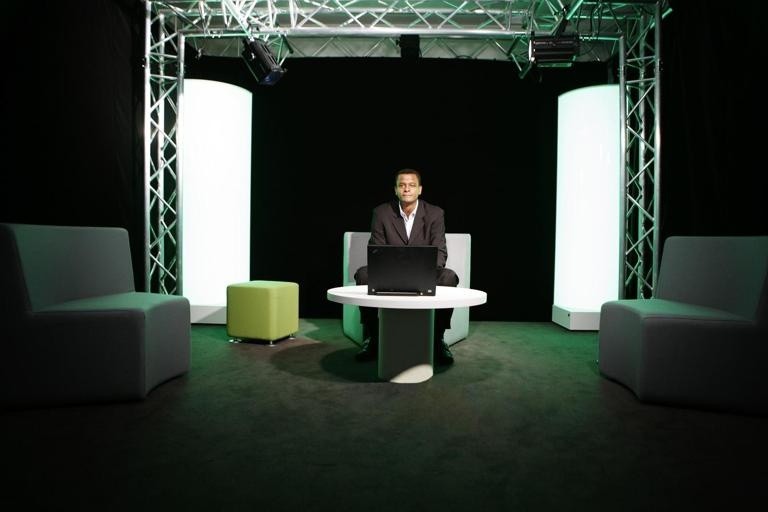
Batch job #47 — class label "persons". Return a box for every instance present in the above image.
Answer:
[353,168,460,371]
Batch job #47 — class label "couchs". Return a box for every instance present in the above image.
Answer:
[2,225,191,402]
[341,230,474,352]
[597,234,766,407]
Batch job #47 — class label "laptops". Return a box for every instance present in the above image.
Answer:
[367,245,438,295]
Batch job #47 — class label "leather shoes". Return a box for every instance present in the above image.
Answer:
[356,340,377,358]
[435,341,454,366]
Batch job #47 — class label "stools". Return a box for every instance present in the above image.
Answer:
[224,278,302,347]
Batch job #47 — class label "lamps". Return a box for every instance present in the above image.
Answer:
[526,4,580,65]
[236,19,295,89]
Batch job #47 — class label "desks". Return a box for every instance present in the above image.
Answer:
[328,284,487,387]
